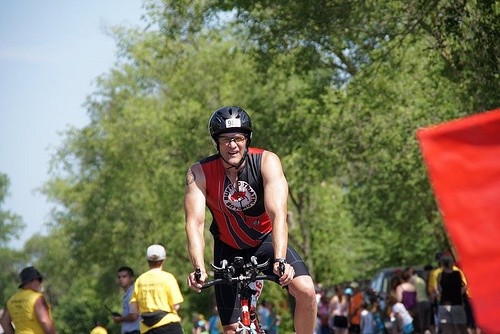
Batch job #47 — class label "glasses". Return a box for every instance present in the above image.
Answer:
[217,136,245,143]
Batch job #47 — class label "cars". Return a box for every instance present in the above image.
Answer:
[371,266,429,302]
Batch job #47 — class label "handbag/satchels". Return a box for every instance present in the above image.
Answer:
[140,310,169,326]
[334,315,347,328]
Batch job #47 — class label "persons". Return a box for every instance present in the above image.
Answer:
[0,244,468,334]
[183,106,318,334]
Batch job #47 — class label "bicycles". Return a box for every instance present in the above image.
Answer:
[192,255,290,334]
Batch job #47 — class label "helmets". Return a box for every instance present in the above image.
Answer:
[209,106,252,138]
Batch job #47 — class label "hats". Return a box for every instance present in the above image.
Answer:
[146,246,165,261]
[17,266,44,287]
